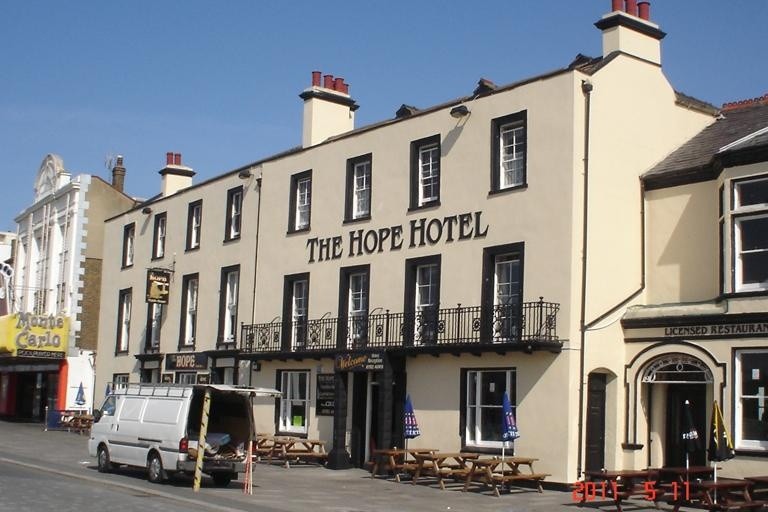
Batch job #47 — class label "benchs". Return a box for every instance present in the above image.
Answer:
[569,480,768,512]
[256,447,328,469]
[366,460,552,498]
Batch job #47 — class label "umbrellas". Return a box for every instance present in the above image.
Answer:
[707,399,737,503]
[400,393,420,460]
[500,390,520,476]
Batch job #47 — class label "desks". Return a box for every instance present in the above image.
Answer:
[371,448,441,483]
[578,466,722,512]
[411,454,480,490]
[675,475,768,512]
[463,456,544,497]
[267,438,330,469]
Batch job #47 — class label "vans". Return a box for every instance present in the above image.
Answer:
[86,381,286,485]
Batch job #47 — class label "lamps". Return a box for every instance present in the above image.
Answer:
[451,104,471,118]
